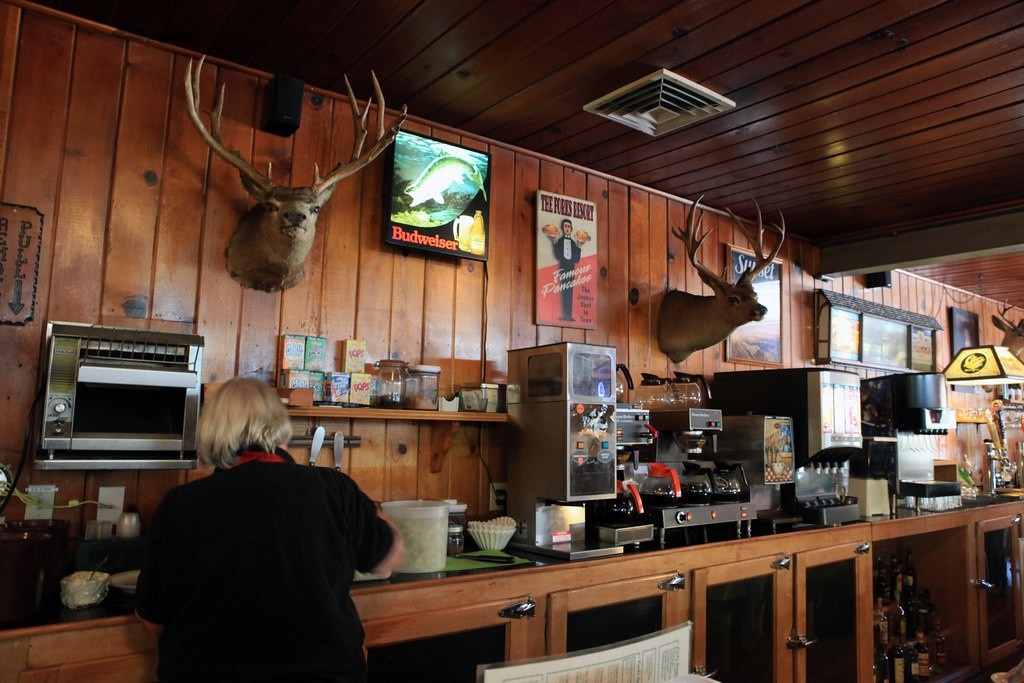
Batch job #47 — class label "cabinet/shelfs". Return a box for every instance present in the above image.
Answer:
[0,499,1024,683]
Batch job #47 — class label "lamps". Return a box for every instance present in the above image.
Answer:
[940,273,1024,386]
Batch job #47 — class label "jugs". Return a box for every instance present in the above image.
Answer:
[634,373,679,411]
[639,463,681,507]
[672,371,712,410]
[679,461,717,506]
[596,480,645,528]
[712,463,749,503]
[590,360,634,402]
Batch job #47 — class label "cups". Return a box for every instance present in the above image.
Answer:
[86,521,112,541]
[953,392,988,420]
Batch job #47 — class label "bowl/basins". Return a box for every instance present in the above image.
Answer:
[468,517,516,550]
[61,574,109,608]
[116,514,140,537]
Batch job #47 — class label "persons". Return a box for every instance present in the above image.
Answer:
[135,376,404,683]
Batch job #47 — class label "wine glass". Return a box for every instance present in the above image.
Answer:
[1005,409,1023,430]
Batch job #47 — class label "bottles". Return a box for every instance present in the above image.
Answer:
[874,632,930,683]
[934,624,948,676]
[874,550,916,606]
[449,526,464,555]
[1008,388,1022,400]
[375,360,405,408]
[874,593,943,650]
[406,365,441,410]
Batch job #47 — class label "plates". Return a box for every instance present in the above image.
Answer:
[111,571,140,593]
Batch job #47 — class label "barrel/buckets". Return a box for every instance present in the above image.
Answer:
[380,500,449,573]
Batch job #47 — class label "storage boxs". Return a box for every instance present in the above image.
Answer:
[439,396,459,412]
[201,333,369,407]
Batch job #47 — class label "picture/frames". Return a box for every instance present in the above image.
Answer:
[725,244,783,367]
[951,307,980,392]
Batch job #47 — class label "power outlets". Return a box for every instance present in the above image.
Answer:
[490,483,506,510]
